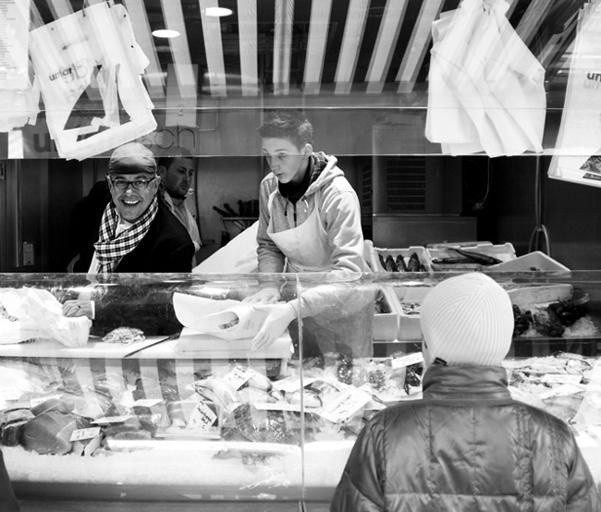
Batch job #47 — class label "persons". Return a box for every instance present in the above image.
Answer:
[250,108,377,357]
[328,269,601,512]
[158,145,203,268]
[59,142,195,336]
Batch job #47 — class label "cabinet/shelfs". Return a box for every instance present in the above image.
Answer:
[0,271,601,511]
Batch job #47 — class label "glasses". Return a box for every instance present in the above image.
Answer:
[106,178,155,190]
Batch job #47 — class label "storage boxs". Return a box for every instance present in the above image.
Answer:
[373,246,431,281]
[386,281,435,340]
[427,240,516,280]
[483,251,573,289]
[300,281,398,341]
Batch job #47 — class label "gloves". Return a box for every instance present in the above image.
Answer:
[251,304,294,351]
[242,288,280,303]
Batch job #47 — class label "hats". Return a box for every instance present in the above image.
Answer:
[418,272,514,365]
[107,142,158,174]
